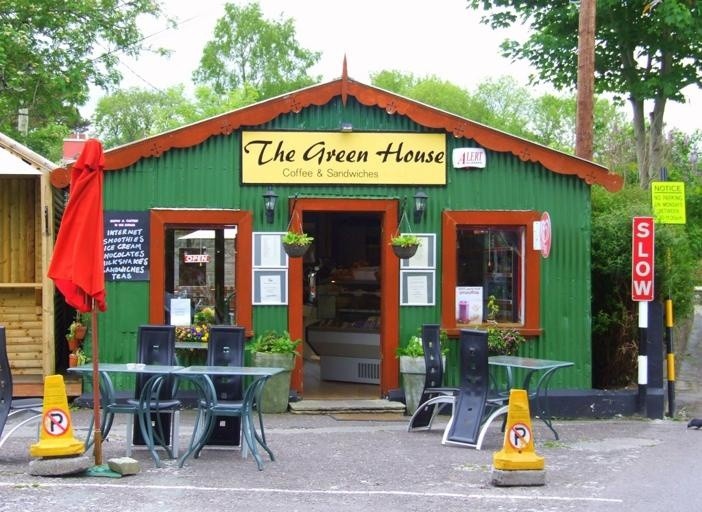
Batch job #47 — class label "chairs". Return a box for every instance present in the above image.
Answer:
[126,325,181,459]
[441,329,509,450]
[193,326,251,459]
[407,324,461,433]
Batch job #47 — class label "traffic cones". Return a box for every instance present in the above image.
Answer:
[494,389,545,470]
[30,374,86,456]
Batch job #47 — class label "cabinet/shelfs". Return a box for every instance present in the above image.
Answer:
[305,266,380,385]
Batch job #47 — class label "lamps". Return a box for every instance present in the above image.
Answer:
[261,191,279,225]
[413,191,433,224]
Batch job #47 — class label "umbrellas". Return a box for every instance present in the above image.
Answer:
[45,136,110,469]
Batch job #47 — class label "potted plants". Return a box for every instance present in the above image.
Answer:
[392,328,450,415]
[244,329,304,413]
[281,232,316,258]
[66,321,88,368]
[388,234,422,260]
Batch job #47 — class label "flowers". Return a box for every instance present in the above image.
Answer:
[485,327,527,353]
[174,323,209,342]
[194,306,216,323]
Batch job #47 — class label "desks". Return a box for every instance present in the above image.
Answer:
[67,362,185,468]
[172,366,285,471]
[487,355,574,439]
